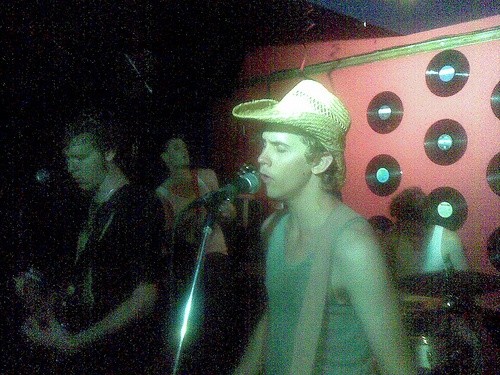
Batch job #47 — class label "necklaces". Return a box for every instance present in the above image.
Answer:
[87,173,129,227]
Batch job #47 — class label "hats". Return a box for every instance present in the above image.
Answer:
[232,80,351,193]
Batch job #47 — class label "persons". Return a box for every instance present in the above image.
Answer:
[376,188,495,375]
[13,106,158,375]
[230,78,418,375]
[154,133,237,375]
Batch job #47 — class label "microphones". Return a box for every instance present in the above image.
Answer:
[188,172,262,210]
